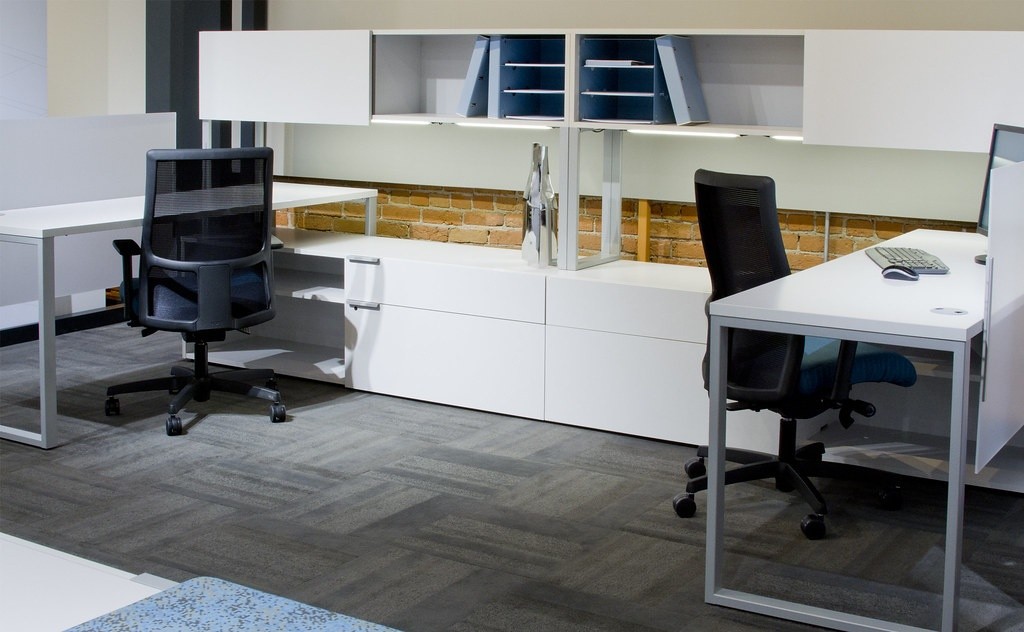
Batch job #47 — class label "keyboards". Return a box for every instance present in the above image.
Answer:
[865,247,950,273]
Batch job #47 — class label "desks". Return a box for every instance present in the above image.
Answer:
[0,181,378,449]
[704,229,1024,632]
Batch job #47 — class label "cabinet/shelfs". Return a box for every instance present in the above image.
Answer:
[199,29,1024,154]
[183,227,1024,493]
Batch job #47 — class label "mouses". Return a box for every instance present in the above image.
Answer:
[882,265,920,281]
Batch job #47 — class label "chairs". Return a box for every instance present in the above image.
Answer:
[105,147,286,436]
[673,169,917,540]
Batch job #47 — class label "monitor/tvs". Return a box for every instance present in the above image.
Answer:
[974,124,1024,267]
